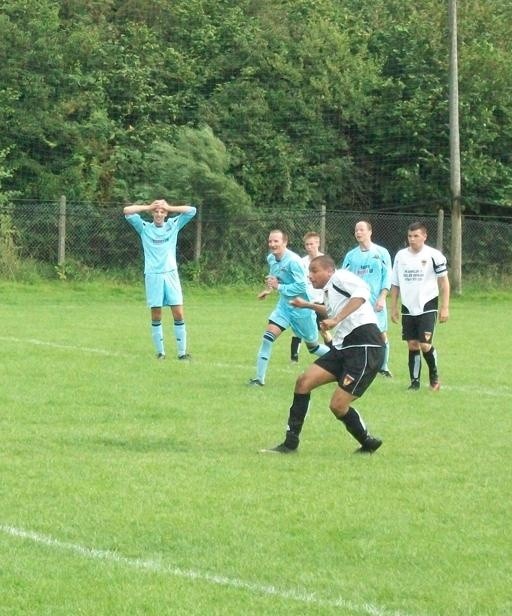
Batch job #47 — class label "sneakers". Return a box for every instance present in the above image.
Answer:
[256,443,296,454]
[353,438,382,454]
[246,378,263,386]
[408,382,420,391]
[429,382,439,390]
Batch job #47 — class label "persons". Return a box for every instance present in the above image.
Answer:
[287,231,330,361]
[249,229,330,386]
[264,255,383,454]
[389,221,450,391]
[340,221,394,379]
[123,199,197,362]
